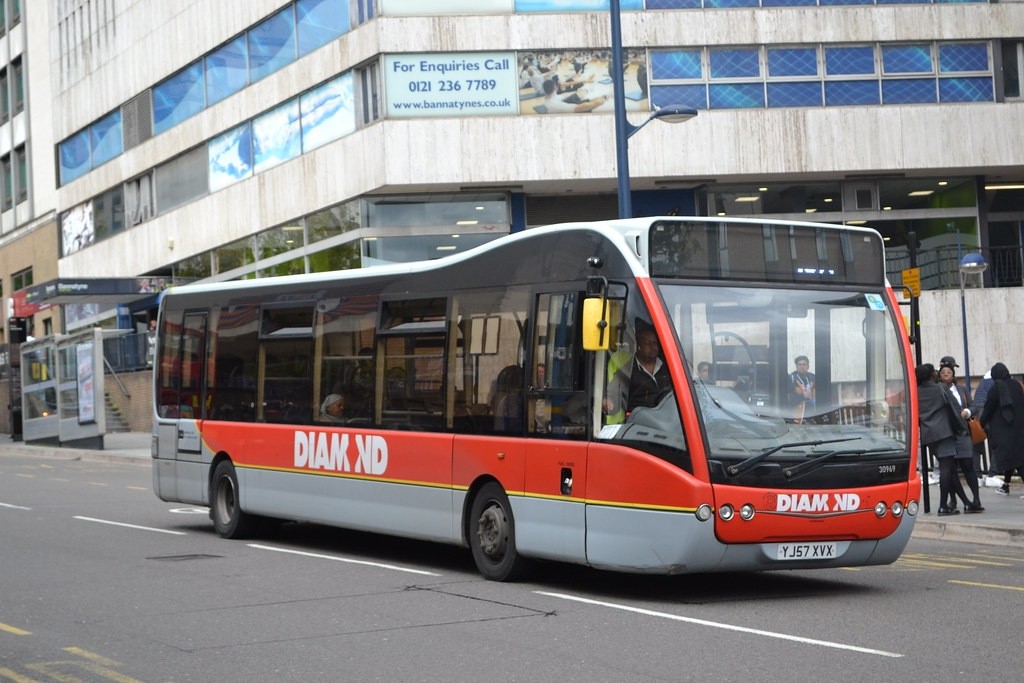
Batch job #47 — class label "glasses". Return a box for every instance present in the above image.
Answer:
[940,371,952,375]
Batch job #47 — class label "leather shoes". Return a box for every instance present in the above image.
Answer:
[964,503,982,514]
[938,507,960,516]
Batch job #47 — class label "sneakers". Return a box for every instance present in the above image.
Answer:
[996,484,1010,496]
[1020,495,1024,499]
[977,474,1004,487]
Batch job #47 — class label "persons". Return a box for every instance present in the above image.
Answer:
[207,347,408,428]
[938,356,1024,509]
[914,363,985,516]
[787,356,816,424]
[536,363,545,431]
[61,200,93,255]
[26,331,36,356]
[693,361,715,386]
[517,50,649,115]
[602,327,673,424]
[150,320,157,330]
[139,278,149,293]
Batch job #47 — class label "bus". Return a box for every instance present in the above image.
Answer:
[150,214,923,585]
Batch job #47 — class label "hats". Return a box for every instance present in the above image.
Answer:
[940,356,959,368]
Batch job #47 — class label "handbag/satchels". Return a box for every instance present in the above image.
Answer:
[968,416,987,445]
[938,382,967,437]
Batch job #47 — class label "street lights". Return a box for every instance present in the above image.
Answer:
[615,103,699,220]
[959,253,988,397]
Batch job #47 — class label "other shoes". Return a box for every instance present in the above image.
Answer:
[947,503,957,508]
[973,501,981,507]
